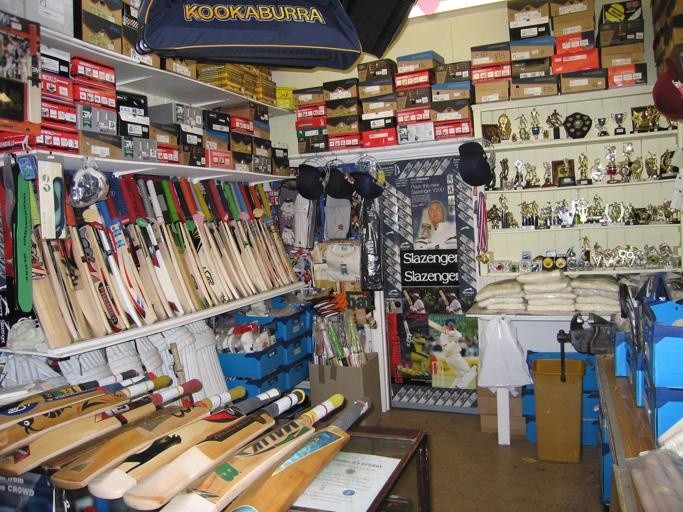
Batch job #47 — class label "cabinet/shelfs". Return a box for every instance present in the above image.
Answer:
[0,24,295,185]
[472,83,682,277]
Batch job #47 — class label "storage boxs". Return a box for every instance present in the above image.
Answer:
[0,0,299,112]
[522,350,598,444]
[216,303,312,400]
[614,297,683,448]
[292,49,471,152]
[472,1,647,105]
[0,43,289,175]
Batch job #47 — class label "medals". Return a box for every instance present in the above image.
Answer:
[475,192,489,263]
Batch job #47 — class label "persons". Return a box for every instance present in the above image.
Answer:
[444,292,464,315]
[439,318,477,389]
[396,328,431,377]
[414,199,458,251]
[409,292,426,314]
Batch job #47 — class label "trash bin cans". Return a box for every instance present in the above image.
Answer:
[533,358,586,463]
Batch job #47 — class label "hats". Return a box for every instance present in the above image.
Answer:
[411,293,422,298]
[445,318,457,325]
[448,293,457,299]
[652,58,683,120]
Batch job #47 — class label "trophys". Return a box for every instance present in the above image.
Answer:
[486,193,682,226]
[488,235,674,273]
[484,105,679,191]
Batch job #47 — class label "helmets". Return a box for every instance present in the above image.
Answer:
[355,155,385,199]
[458,137,496,186]
[327,159,356,199]
[297,156,331,200]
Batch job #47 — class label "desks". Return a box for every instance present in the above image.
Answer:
[464,302,620,445]
[1,397,434,510]
[595,353,682,512]
